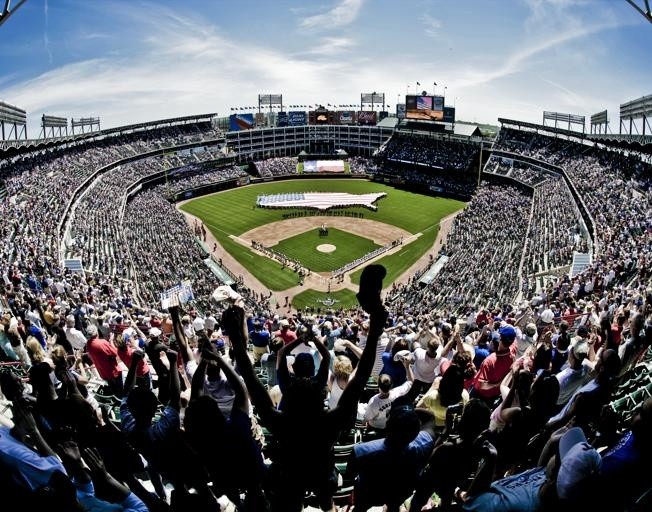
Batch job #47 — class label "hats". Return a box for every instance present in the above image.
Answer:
[526,323,537,334]
[122,327,162,339]
[497,324,516,342]
[389,396,420,441]
[556,427,603,500]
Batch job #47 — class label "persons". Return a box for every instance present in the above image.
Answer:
[379,128,650,329]
[335,273,344,284]
[1,296,649,511]
[254,156,298,177]
[282,209,364,218]
[327,284,330,293]
[1,124,272,321]
[348,155,379,174]
[250,240,311,286]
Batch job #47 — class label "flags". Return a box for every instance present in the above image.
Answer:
[303,159,345,173]
[416,82,420,86]
[434,82,437,86]
